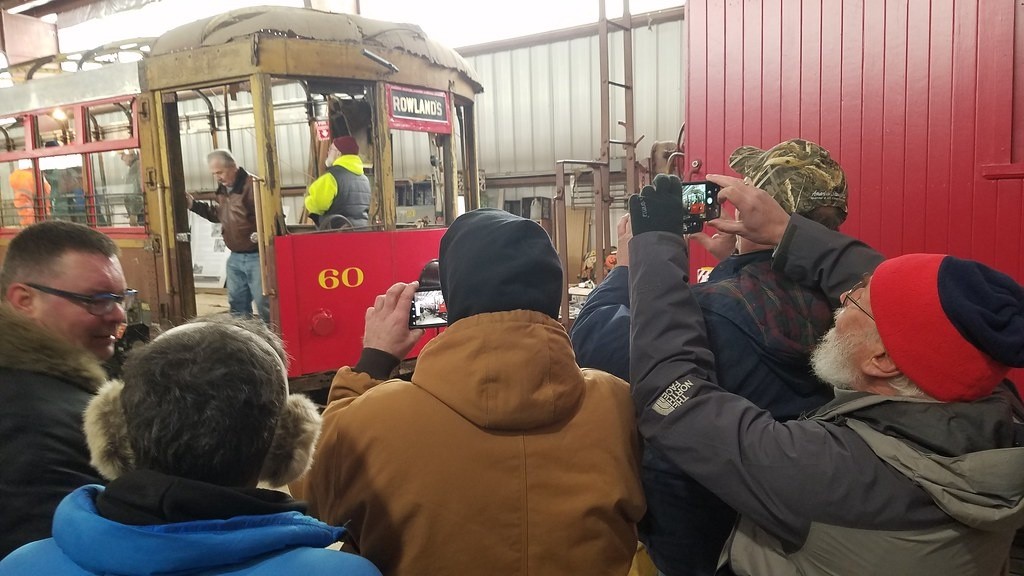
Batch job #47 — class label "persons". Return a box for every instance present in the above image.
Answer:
[0,315,381,576]
[570,139,850,576]
[420,304,425,321]
[10,161,51,225]
[628,173,1024,576]
[305,136,371,230]
[289,207,648,576]
[0,220,137,562]
[185,149,271,326]
[118,148,145,226]
[42,141,76,220]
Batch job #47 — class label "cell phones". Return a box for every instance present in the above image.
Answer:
[682,221,704,234]
[406,285,448,329]
[681,182,721,222]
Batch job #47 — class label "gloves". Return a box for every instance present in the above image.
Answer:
[628,172,684,237]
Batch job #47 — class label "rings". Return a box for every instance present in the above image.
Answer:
[745,182,749,185]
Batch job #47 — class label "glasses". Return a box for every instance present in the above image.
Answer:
[839,273,875,326]
[23,283,138,317]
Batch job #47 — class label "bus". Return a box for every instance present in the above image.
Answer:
[424,299,450,321]
[681,189,705,214]
[0,2,485,396]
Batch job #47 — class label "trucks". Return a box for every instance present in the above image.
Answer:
[605,0,1023,402]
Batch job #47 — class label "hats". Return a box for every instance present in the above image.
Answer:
[727,140,849,216]
[869,253,1024,404]
[333,136,359,155]
